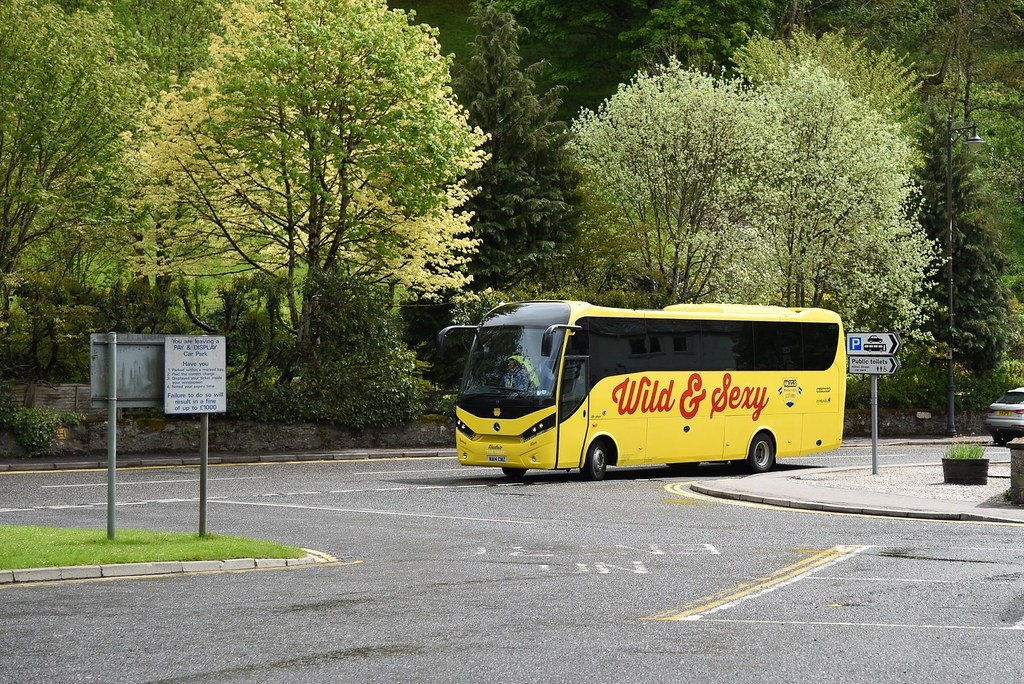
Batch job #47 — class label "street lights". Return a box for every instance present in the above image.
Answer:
[946,109,981,434]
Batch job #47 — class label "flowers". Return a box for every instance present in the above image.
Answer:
[940,431,993,458]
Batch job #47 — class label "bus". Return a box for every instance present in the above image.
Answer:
[437,299,847,480]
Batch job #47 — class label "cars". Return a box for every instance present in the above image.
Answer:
[985,387,1024,443]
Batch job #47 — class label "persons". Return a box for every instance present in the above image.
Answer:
[499,359,529,390]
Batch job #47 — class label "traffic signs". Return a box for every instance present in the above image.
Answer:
[847,331,904,356]
[848,356,901,375]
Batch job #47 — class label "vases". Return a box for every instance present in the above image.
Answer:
[941,458,990,486]
